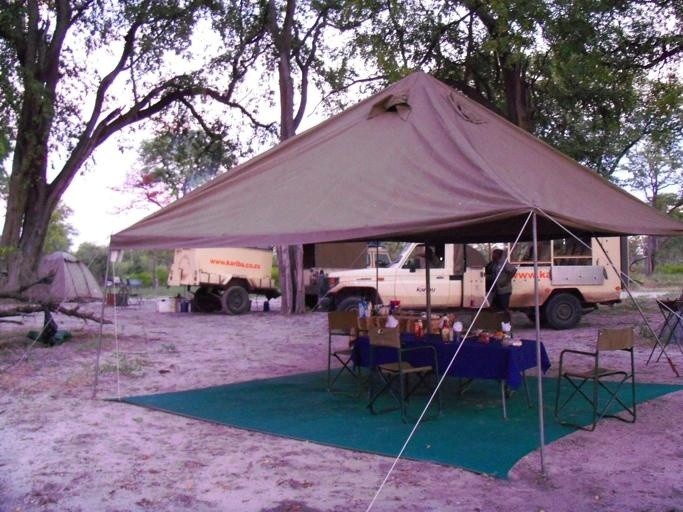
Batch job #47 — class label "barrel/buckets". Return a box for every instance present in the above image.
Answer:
[158,294,188,314]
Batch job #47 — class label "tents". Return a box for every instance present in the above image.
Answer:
[19,251,105,303]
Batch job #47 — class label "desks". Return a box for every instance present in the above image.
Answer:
[355,334,542,419]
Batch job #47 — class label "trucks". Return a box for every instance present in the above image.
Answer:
[314,226,634,332]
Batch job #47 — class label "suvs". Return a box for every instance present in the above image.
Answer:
[288,241,395,306]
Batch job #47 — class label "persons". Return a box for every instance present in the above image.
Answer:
[484,248,517,331]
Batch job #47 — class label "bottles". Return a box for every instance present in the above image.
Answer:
[359,295,463,345]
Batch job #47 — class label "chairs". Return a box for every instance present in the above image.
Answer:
[326,308,442,424]
[553,326,636,433]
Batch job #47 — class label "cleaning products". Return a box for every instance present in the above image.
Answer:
[385,310,399,329]
[453,318,463,343]
[438,313,449,337]
